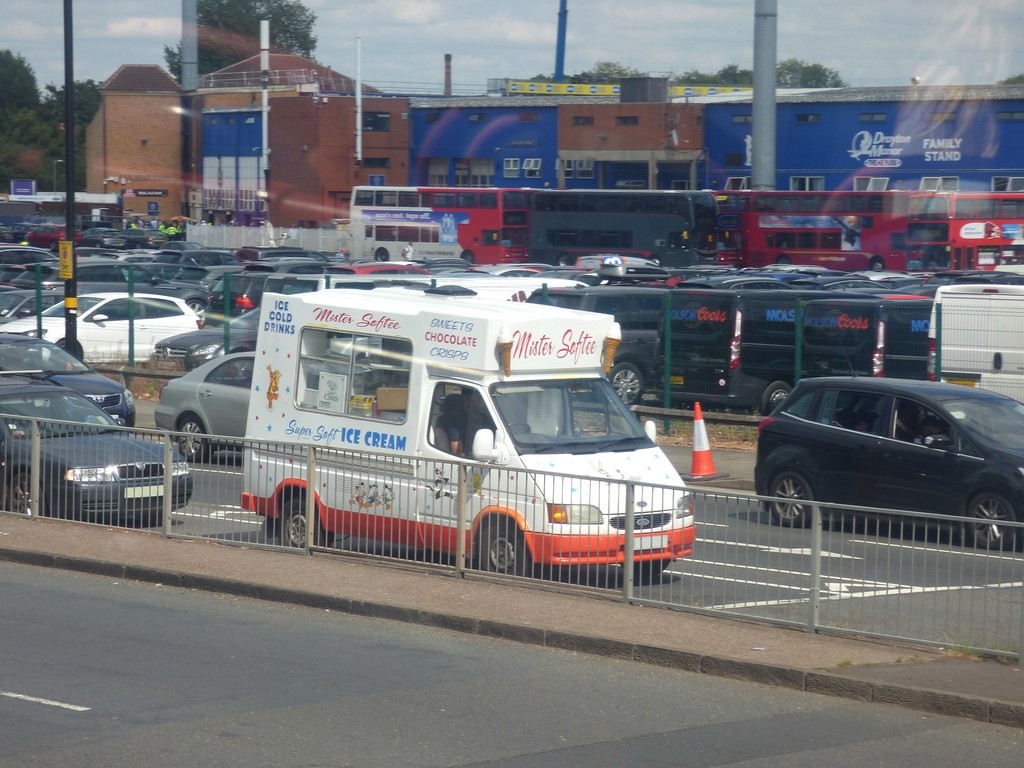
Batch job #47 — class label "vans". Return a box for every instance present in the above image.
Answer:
[238,286,696,582]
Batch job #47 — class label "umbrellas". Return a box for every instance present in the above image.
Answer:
[168,215,193,222]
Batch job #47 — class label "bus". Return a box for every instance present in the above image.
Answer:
[699,190,924,273]
[349,184,533,267]
[521,185,720,267]
[904,191,1024,273]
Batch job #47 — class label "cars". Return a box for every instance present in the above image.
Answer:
[0,385,195,527]
[0,332,136,436]
[0,217,1024,410]
[153,351,256,464]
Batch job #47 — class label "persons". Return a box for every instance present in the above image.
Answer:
[913,415,942,450]
[402,241,415,261]
[335,248,346,258]
[852,413,871,433]
[127,215,213,241]
[448,383,498,459]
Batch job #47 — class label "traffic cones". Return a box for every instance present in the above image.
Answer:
[681,400,732,482]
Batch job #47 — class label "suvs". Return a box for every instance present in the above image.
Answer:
[753,373,1024,552]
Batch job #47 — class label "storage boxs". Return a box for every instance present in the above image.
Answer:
[376,387,408,411]
[305,362,388,419]
